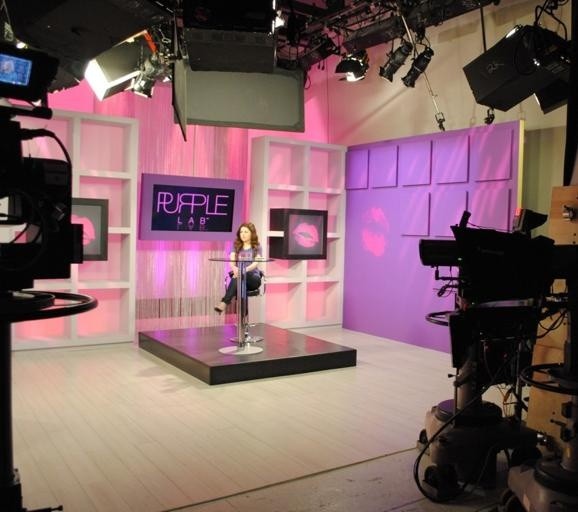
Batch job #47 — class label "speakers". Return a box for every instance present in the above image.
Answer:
[183,7,273,73]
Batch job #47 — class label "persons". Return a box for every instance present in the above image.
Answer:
[213,222,264,341]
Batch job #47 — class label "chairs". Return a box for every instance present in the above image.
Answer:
[223,269,267,344]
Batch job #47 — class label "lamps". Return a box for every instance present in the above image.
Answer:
[174,1,303,142]
[463,0,572,114]
[84,32,170,102]
[381,42,412,85]
[333,51,369,87]
[402,46,436,88]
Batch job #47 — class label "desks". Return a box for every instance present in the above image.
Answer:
[208,257,276,356]
